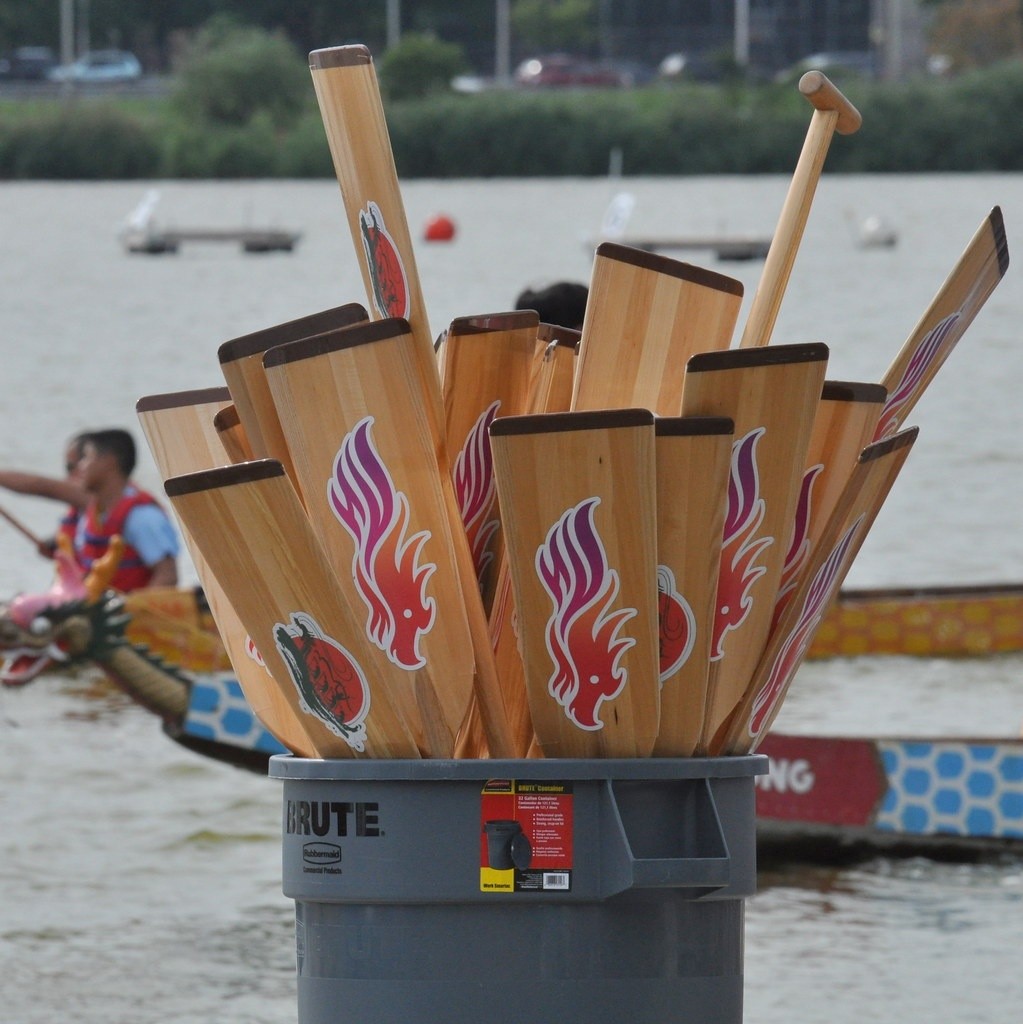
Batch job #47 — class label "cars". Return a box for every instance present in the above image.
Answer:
[453,48,867,95]
[0,45,141,89]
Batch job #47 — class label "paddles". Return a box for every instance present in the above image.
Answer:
[130,38,1015,762]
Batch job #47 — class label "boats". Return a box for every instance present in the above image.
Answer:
[123,220,304,256]
[589,234,772,261]
[53,556,1022,873]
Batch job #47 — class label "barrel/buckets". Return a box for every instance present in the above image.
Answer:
[267,744,770,1024]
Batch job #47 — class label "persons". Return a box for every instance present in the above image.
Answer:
[40,431,137,566]
[0,427,181,606]
